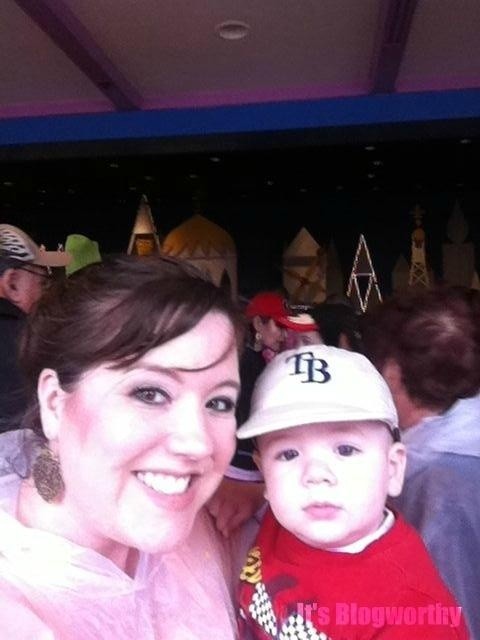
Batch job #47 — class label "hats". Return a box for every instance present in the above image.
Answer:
[233,343,402,444]
[0,222,72,269]
[244,292,291,323]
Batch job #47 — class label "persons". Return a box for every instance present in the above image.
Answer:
[0,222,479,639]
